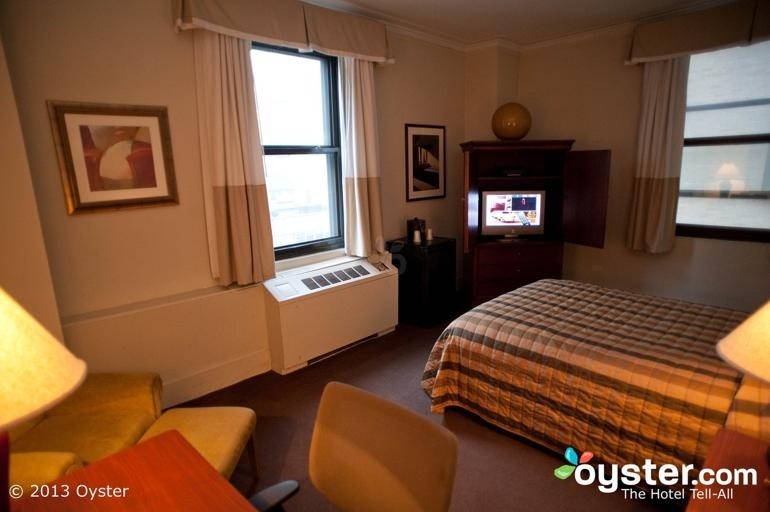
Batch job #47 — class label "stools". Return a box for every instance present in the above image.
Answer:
[307,382,457,511]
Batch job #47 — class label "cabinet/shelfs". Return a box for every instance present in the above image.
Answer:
[459,139,611,309]
[386,236,456,329]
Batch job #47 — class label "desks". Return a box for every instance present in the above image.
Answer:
[12,430,260,511]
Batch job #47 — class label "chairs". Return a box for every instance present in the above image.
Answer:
[11,373,258,498]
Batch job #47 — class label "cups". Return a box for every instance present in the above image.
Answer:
[414,230,421,242]
[427,228,433,241]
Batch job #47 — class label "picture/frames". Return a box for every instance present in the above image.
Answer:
[404,123,447,202]
[46,98,178,214]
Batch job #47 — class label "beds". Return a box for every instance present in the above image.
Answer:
[421,278,769,496]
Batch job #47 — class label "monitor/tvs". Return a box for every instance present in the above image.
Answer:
[480,190,545,241]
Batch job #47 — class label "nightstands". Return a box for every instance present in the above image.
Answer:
[684,428,769,510]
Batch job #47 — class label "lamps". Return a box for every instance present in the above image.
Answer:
[0,288,88,510]
[715,302,769,384]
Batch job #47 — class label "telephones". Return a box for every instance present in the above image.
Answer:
[385,240,404,253]
